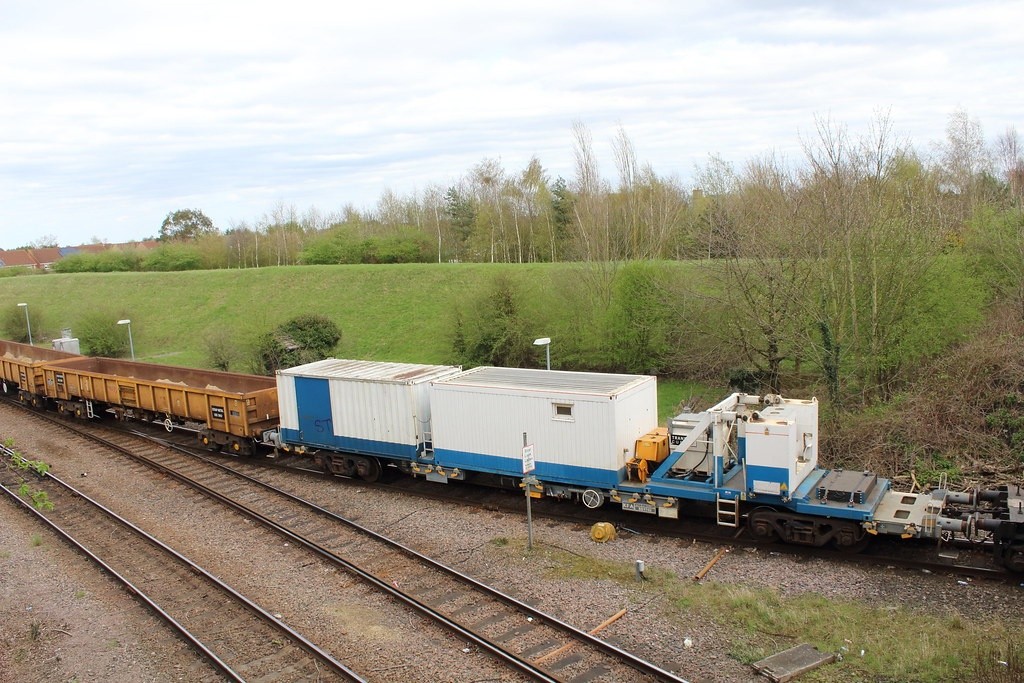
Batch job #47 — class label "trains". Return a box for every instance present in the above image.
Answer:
[0,338,1024,581]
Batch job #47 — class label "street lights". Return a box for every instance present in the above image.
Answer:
[17,302,33,346]
[117,320,135,361]
[533,338,550,370]
